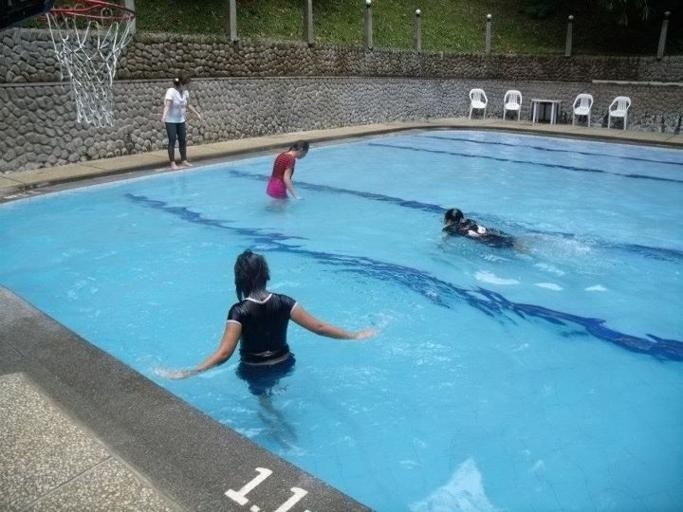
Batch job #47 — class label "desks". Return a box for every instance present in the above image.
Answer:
[530,98,562,126]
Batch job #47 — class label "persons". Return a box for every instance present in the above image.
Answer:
[441,208,536,255]
[265,139,307,200]
[160,74,201,169]
[146,247,377,449]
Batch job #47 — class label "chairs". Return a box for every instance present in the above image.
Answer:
[607,95,631,130]
[502,89,523,122]
[571,93,593,127]
[468,88,488,120]
